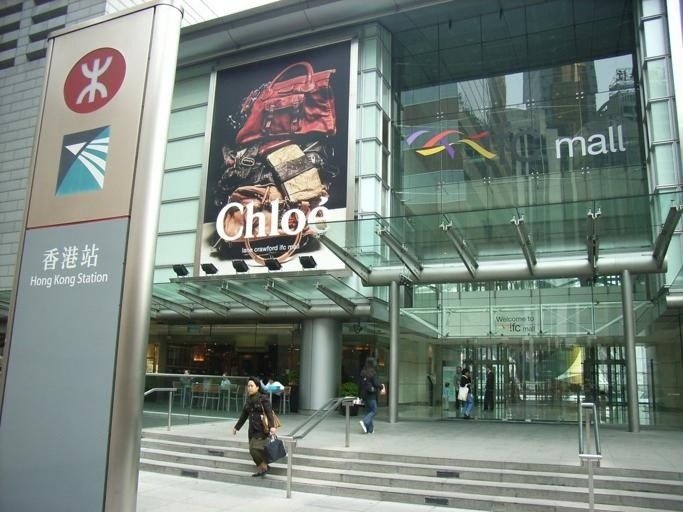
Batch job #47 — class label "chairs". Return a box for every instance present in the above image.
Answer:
[171,381,291,416]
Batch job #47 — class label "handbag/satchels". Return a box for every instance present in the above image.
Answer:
[258,396,282,434]
[457,383,469,402]
[262,431,286,464]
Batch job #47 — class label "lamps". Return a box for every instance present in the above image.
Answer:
[172,256,318,278]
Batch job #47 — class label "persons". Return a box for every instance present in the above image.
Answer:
[453,366,463,408]
[232,376,286,477]
[259,377,284,398]
[456,368,473,418]
[358,356,385,434]
[178,374,200,409]
[481,367,493,410]
[442,382,449,411]
[219,375,231,395]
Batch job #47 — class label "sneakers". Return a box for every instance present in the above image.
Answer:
[251,464,271,478]
[359,418,368,433]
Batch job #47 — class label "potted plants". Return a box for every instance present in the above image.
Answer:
[338,381,359,416]
